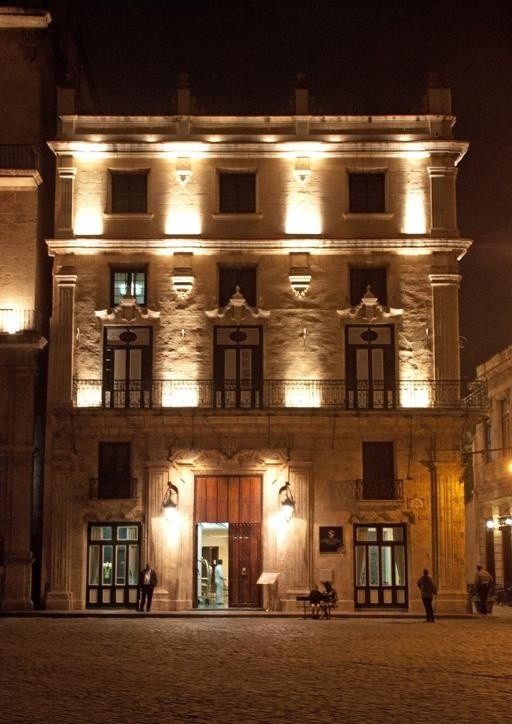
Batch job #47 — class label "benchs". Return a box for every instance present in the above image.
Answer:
[296,595,334,620]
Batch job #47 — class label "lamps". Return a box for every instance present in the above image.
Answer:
[161,492,178,521]
[280,497,296,520]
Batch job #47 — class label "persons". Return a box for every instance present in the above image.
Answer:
[309,585,328,619]
[417,569,437,623]
[322,584,337,618]
[321,530,342,552]
[214,560,226,604]
[141,563,158,612]
[475,565,494,615]
